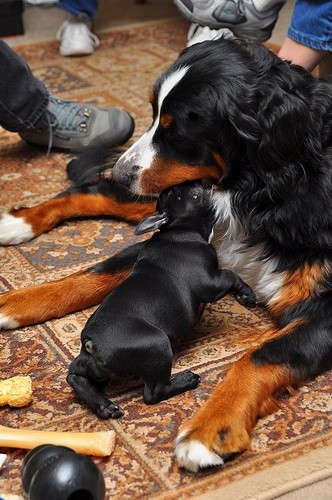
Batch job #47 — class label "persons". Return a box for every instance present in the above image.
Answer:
[56,0,100,56]
[0,40,135,158]
[174,0,332,75]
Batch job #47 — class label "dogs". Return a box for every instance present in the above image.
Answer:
[0,33,332,474]
[66,175,258,421]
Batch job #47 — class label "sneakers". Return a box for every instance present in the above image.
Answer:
[19,91,135,158]
[173,0,287,44]
[56,19,100,57]
